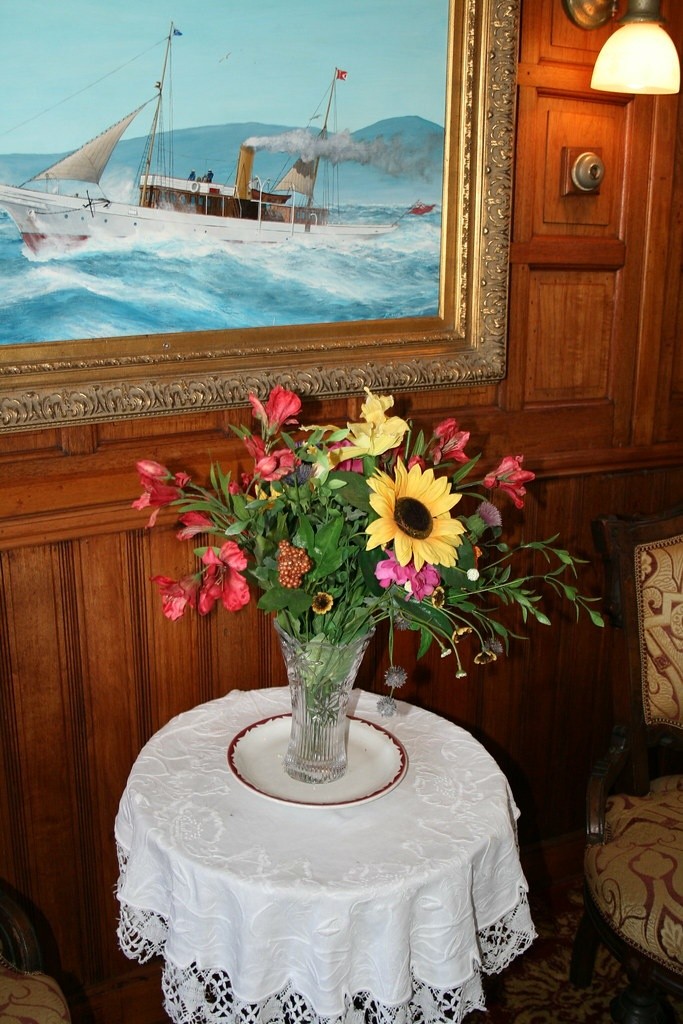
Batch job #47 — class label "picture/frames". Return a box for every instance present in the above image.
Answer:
[0,0,517,434]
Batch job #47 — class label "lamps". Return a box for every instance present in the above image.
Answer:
[563,0,680,96]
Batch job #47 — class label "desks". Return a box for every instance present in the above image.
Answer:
[114,683,538,1024]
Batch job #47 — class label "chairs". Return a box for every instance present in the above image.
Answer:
[0,877,95,1023]
[568,509,683,1024]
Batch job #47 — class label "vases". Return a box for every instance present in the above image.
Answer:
[270,619,377,785]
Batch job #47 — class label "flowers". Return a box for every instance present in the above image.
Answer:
[133,381,608,754]
